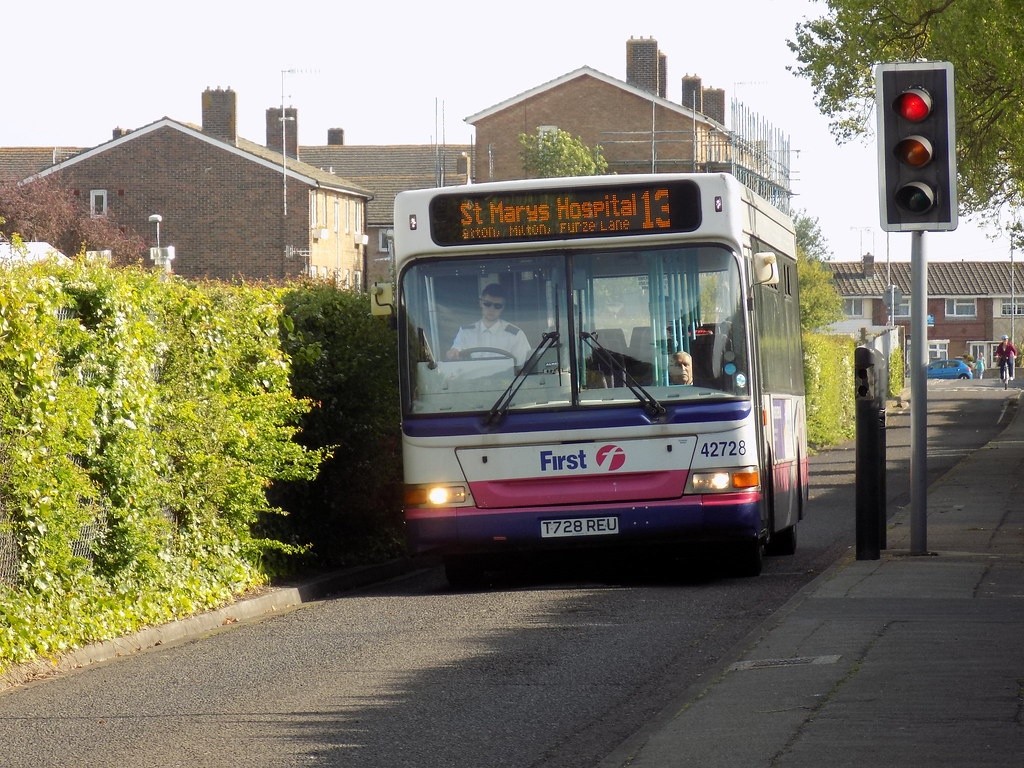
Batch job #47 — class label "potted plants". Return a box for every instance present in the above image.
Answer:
[313,223,329,239]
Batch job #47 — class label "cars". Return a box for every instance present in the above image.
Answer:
[927,360,973,379]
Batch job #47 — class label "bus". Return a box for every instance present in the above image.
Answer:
[368,172,811,577]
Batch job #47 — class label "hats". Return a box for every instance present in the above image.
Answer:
[1002,335,1009,339]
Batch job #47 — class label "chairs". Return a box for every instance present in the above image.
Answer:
[594,328,628,369]
[629,326,653,376]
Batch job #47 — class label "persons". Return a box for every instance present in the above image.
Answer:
[669,351,694,386]
[444,282,530,374]
[638,297,676,385]
[974,352,986,379]
[532,276,582,348]
[993,335,1018,383]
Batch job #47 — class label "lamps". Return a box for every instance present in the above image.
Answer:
[148,214,162,222]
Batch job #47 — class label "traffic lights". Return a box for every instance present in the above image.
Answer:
[874,63,959,232]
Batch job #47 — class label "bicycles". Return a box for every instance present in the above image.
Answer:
[995,355,1015,390]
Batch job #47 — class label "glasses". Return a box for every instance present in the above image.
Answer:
[669,362,691,368]
[482,299,504,309]
